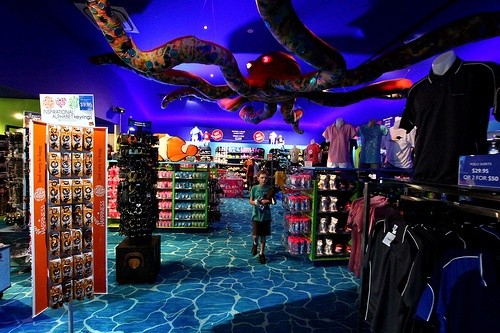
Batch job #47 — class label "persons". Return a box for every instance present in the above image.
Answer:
[400,51,500,183]
[305,139,321,166]
[357,119,386,168]
[190,126,214,142]
[320,138,331,166]
[322,118,357,168]
[289,145,300,166]
[386,116,417,169]
[243,158,260,193]
[249,170,276,263]
[269,132,284,144]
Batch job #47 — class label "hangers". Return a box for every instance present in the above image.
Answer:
[389,200,407,228]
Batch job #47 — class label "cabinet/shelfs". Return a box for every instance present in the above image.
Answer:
[108,168,208,229]
[216,147,362,262]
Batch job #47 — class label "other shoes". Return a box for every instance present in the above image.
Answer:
[251,242,258,256]
[259,253,266,264]
